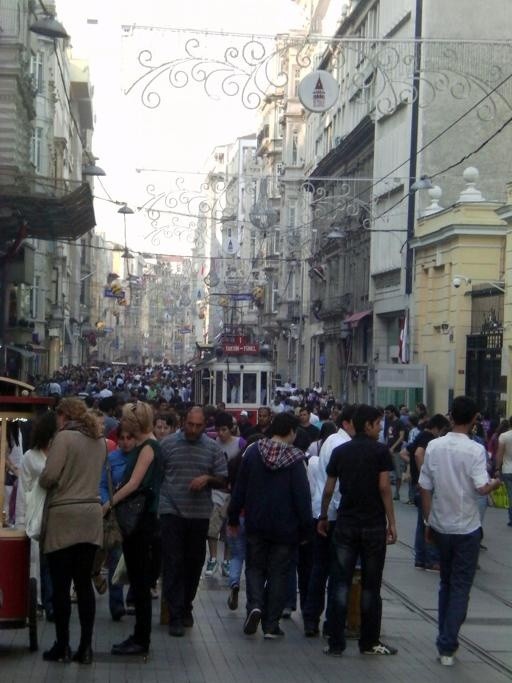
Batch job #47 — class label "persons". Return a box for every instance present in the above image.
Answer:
[314,404,401,656]
[414,395,502,669]
[1,357,511,639]
[100,399,163,657]
[38,394,109,665]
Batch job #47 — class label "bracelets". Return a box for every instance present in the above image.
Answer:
[421,515,430,526]
[317,514,329,520]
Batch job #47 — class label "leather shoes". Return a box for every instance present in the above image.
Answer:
[169,617,185,637]
[70,646,93,663]
[182,611,194,627]
[112,636,149,655]
[44,640,72,663]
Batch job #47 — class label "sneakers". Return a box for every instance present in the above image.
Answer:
[204,560,219,578]
[150,580,159,600]
[221,562,232,577]
[323,626,397,657]
[414,562,440,573]
[441,652,455,665]
[228,582,285,640]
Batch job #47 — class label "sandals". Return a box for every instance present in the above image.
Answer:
[93,571,107,595]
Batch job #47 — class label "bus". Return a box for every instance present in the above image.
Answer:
[190,328,275,424]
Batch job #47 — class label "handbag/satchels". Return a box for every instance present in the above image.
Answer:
[105,510,123,562]
